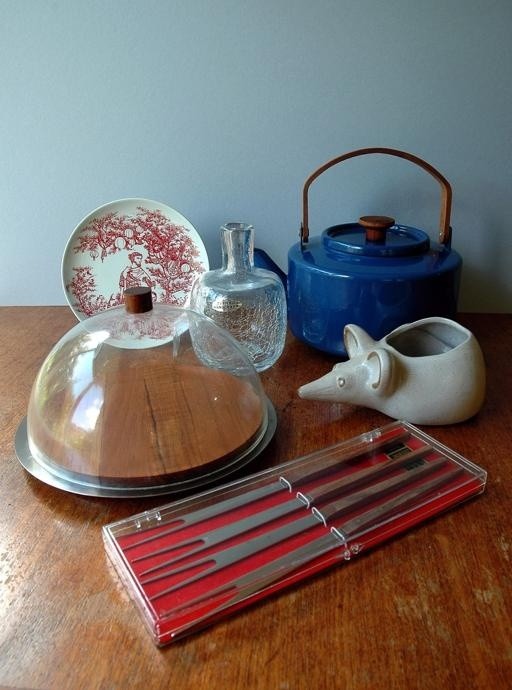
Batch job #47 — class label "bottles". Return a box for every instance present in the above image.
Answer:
[189,222,286,375]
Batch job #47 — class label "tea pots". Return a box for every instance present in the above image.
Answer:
[253,145,463,357]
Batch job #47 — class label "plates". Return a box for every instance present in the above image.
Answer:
[60,197,209,352]
